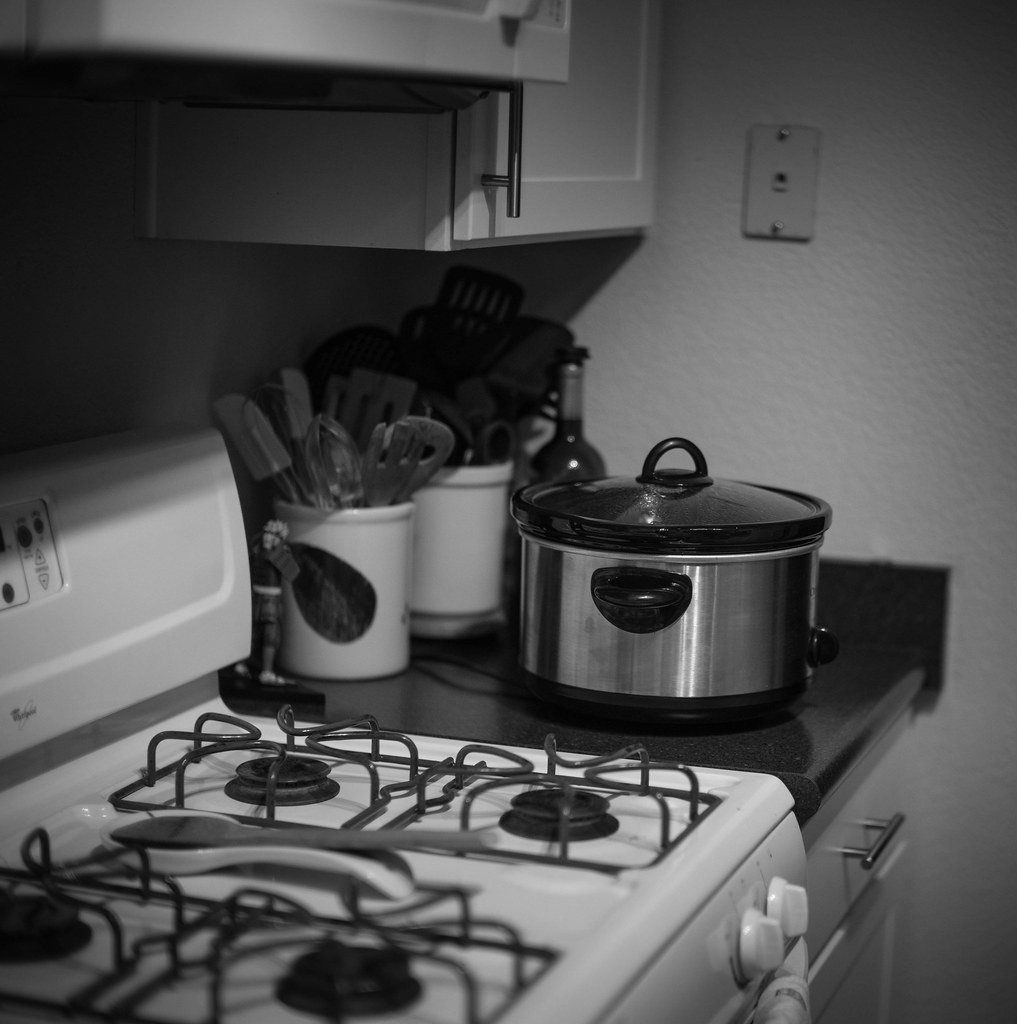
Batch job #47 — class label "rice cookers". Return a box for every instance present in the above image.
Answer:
[497,436,839,726]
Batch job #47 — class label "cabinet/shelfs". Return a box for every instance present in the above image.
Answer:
[799,700,912,1024]
[135,0,660,255]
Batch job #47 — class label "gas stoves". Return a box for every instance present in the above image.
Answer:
[0,420,822,1024]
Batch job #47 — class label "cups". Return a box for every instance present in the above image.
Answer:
[275,496,415,679]
[413,458,513,615]
